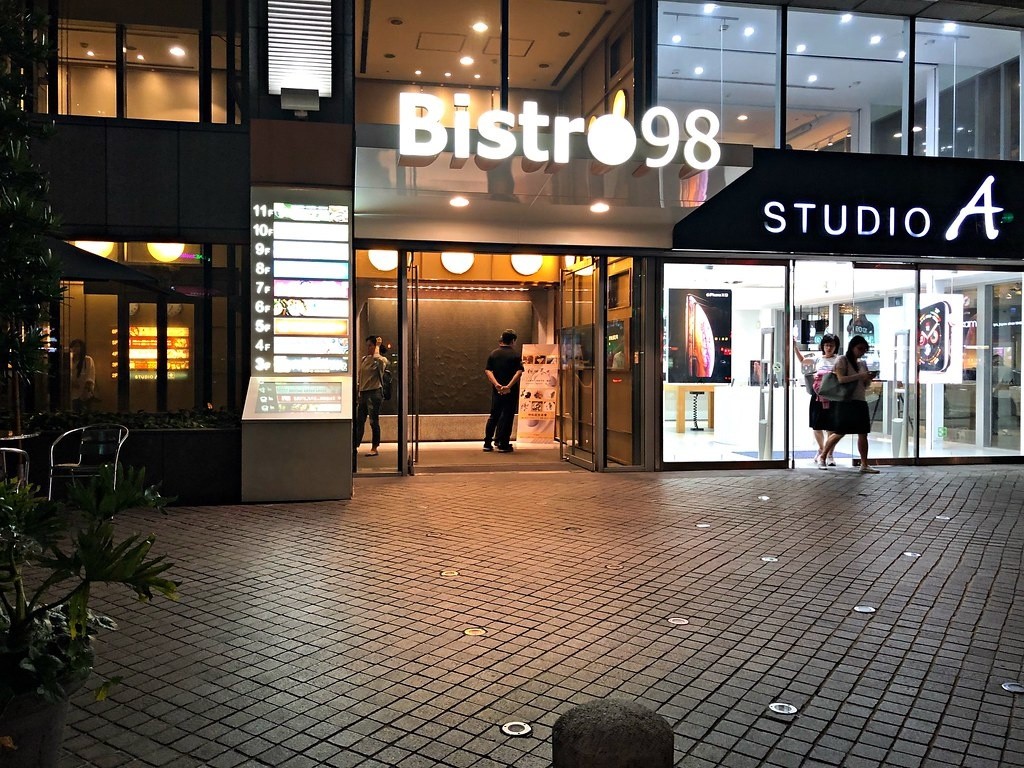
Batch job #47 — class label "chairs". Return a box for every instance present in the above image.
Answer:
[47,423,130,521]
[0,447,30,494]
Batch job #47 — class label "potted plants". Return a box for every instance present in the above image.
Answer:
[0,461,184,768]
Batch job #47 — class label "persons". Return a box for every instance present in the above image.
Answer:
[70,339,95,415]
[356,336,389,456]
[793,334,842,466]
[612,351,624,368]
[483,329,525,451]
[819,336,880,473]
[849,314,874,337]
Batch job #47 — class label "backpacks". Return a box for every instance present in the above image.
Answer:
[363,355,392,401]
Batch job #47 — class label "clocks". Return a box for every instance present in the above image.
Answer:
[130,303,138,316]
[167,304,182,317]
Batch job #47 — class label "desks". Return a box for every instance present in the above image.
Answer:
[664,384,715,434]
[0,428,43,441]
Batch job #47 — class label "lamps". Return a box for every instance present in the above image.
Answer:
[802,127,851,151]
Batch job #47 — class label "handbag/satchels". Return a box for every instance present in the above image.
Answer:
[818,355,855,407]
[804,371,816,395]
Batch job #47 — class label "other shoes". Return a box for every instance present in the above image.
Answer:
[814,449,823,462]
[365,452,378,457]
[493,443,512,447]
[817,457,827,470]
[483,444,494,450]
[497,445,514,452]
[859,465,880,473]
[827,456,836,466]
